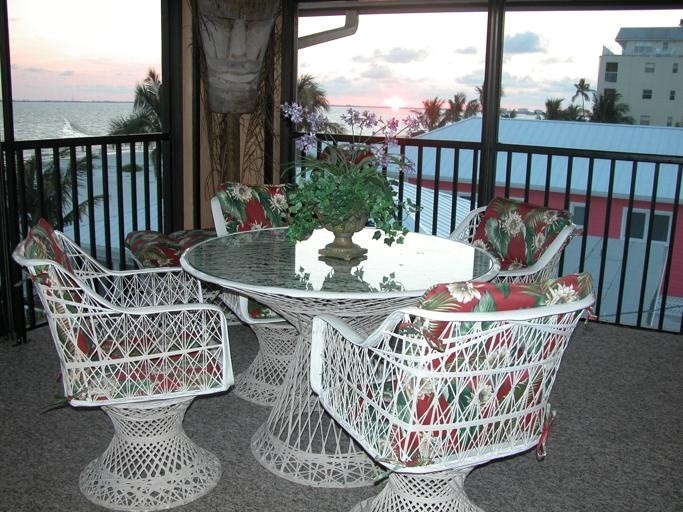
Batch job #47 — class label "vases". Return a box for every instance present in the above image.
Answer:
[315,208,370,263]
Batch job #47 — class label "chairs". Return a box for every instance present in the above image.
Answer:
[13,219,232,511]
[211,183,300,410]
[311,273,598,512]
[448,196,577,285]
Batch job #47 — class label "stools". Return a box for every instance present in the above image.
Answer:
[125,229,245,326]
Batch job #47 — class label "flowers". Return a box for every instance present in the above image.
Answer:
[280,103,423,247]
